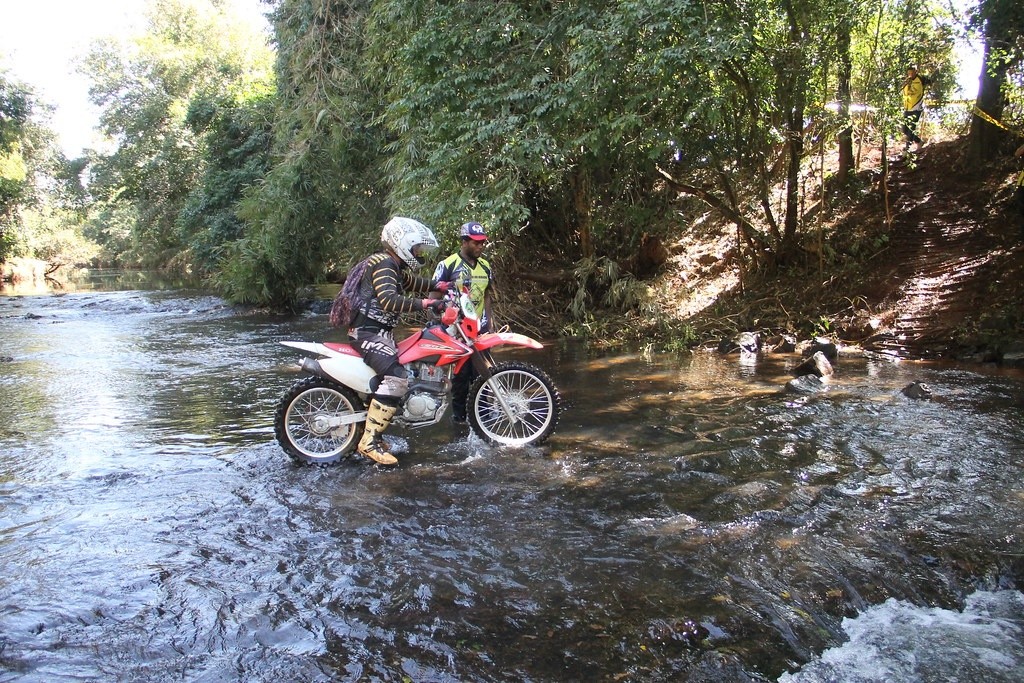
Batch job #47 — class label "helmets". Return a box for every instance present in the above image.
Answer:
[381,217,439,272]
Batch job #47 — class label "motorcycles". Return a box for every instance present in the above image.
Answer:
[273,276,562,467]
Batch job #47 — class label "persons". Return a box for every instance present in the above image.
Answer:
[428,222,501,423]
[901,67,925,151]
[346,216,455,466]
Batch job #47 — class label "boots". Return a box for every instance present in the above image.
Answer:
[358,398,398,465]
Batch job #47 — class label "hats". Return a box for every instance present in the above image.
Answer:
[461,222,487,241]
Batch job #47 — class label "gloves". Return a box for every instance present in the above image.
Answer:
[437,281,455,294]
[423,298,445,315]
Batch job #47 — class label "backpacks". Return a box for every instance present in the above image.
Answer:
[330,256,400,327]
